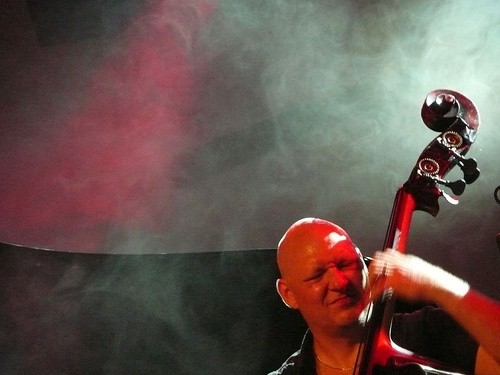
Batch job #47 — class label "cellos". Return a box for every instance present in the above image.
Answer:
[351,89,481,375]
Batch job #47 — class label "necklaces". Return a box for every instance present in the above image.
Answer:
[313,350,357,370]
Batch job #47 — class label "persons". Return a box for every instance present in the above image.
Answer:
[265,218,500,375]
